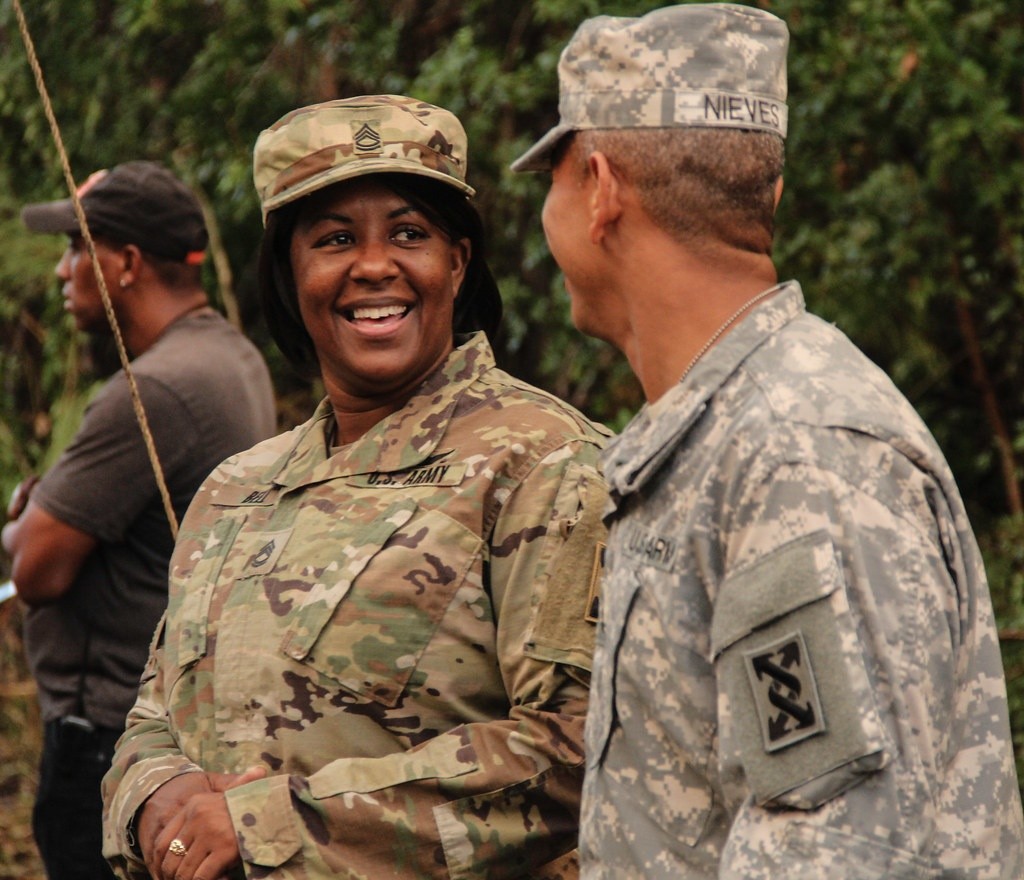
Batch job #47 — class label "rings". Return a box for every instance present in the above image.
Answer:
[169,840,187,856]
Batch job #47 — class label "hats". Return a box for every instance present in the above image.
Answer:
[22,159,208,268]
[511,4,791,173]
[253,95,477,231]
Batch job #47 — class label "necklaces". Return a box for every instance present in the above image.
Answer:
[679,285,781,381]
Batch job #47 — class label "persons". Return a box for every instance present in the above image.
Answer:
[101,93,621,880]
[511,0,1024,879]
[0,161,277,879]
